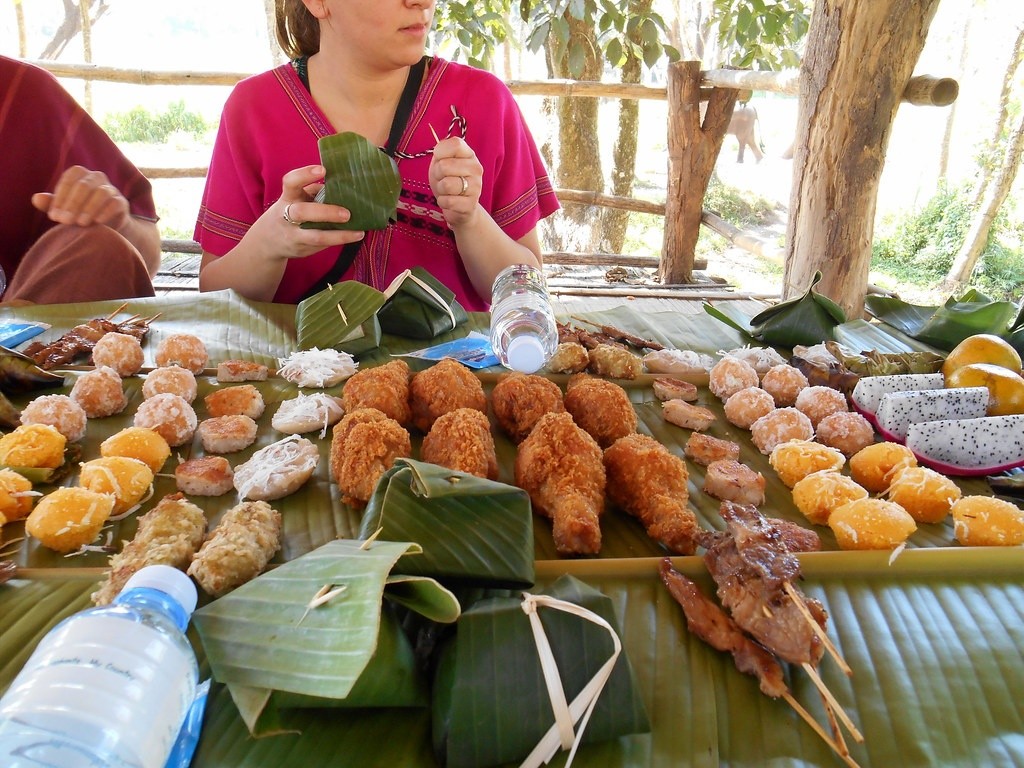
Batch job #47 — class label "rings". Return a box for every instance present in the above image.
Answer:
[458,175,470,196]
[283,202,303,226]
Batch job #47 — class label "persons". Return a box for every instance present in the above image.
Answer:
[192,0,562,318]
[1,54,163,311]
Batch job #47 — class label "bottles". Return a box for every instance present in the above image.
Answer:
[490,265,559,372]
[0,564,199,767]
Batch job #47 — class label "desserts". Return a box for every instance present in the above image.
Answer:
[0,330,207,554]
[767,439,1024,551]
[708,356,874,453]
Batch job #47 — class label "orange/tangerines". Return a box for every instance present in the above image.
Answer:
[940,333,1024,416]
